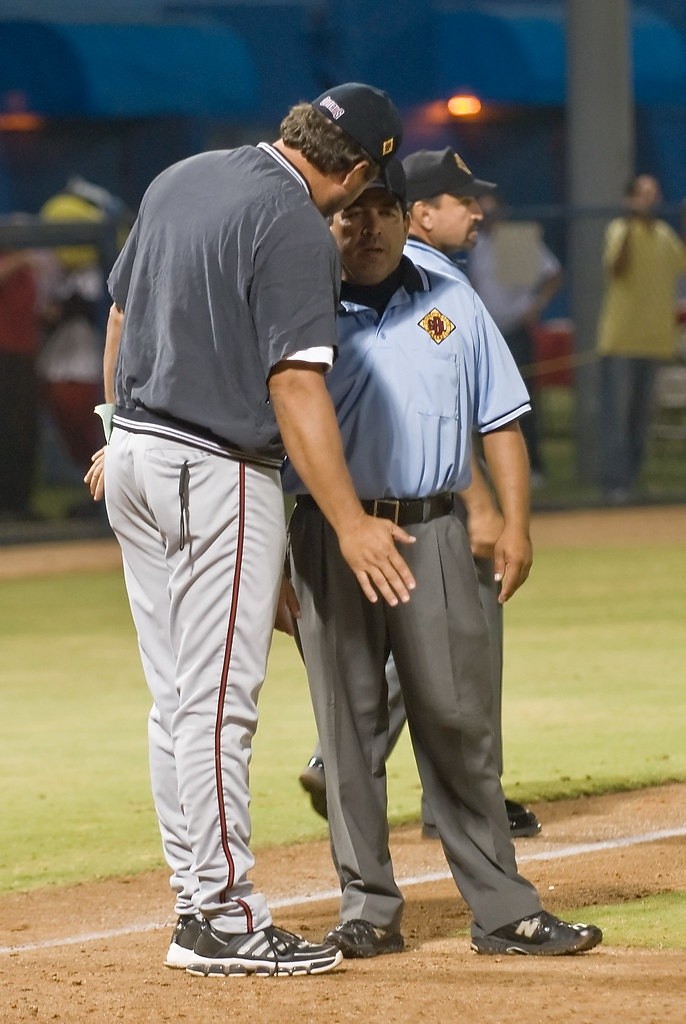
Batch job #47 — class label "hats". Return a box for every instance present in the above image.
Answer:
[312,83,402,197]
[401,146,497,200]
[363,158,407,218]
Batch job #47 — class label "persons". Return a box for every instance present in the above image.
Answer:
[460,169,686,505]
[1,174,136,522]
[85,81,417,979]
[270,156,600,958]
[296,148,543,840]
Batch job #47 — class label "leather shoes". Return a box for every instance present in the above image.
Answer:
[299,757,328,820]
[422,799,541,839]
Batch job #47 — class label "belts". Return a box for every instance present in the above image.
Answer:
[296,492,453,527]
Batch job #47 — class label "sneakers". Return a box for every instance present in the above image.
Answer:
[164,914,202,968]
[470,911,602,956]
[186,917,344,977]
[323,919,404,958]
